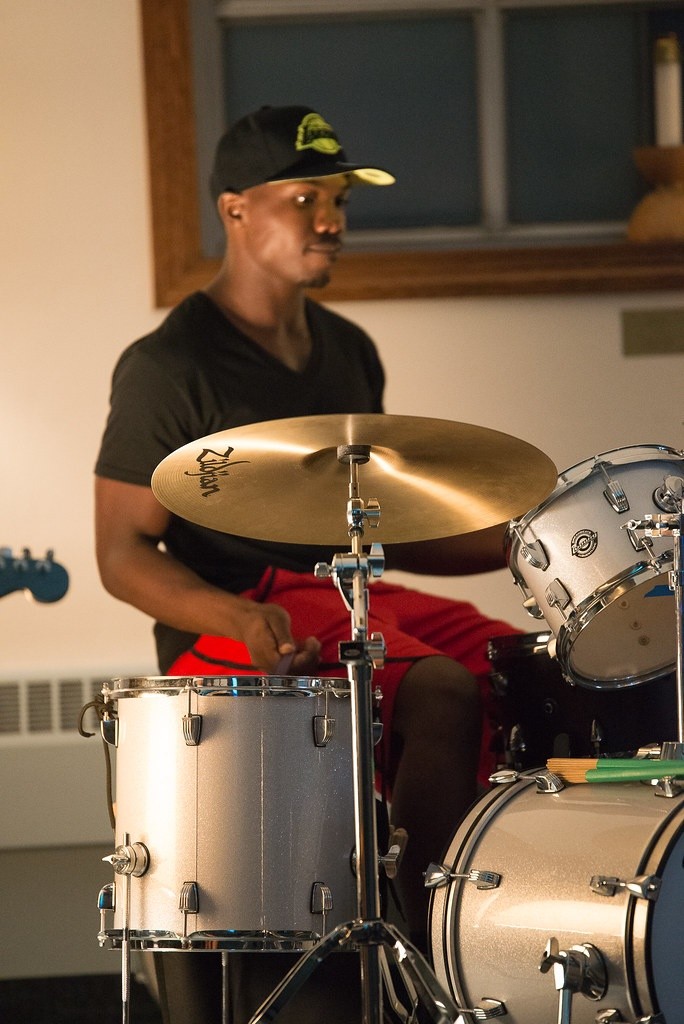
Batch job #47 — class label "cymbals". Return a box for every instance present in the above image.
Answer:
[150,415,555,545]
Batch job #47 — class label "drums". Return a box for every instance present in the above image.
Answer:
[425,756,683,1024]
[499,443,684,692]
[100,675,359,951]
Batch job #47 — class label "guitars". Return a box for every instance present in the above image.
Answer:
[1,546,69,605]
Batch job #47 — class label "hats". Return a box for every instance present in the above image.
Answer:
[207,103,397,201]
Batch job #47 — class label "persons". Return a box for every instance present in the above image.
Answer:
[94,103,528,950]
